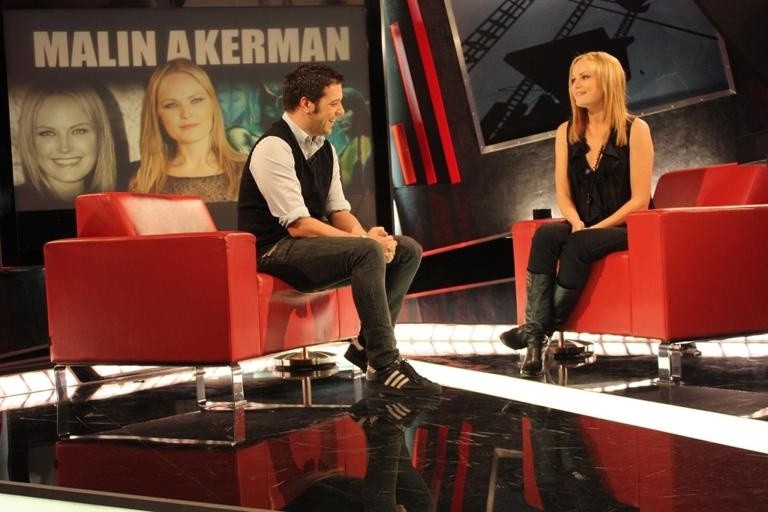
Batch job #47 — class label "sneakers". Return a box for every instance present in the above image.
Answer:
[349,391,441,434]
[344,336,443,398]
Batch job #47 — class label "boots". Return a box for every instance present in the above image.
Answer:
[500,402,579,477]
[500,269,585,379]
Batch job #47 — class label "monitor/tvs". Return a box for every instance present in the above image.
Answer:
[0,0,396,268]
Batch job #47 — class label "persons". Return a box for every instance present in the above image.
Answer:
[499,50,656,379]
[336,87,374,196]
[497,401,640,512]
[13,58,247,211]
[278,392,441,512]
[238,63,442,397]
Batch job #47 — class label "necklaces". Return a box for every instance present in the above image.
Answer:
[581,144,604,204]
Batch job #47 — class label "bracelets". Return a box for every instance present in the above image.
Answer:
[359,234,367,239]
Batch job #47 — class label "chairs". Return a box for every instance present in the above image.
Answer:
[42,193,360,449]
[511,163,768,385]
